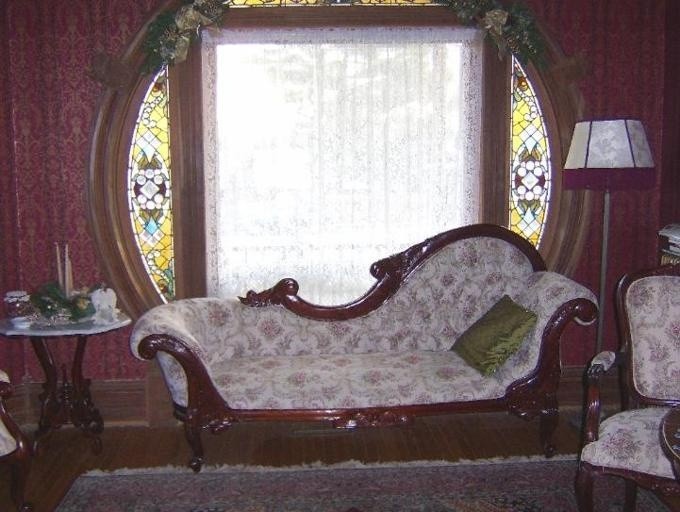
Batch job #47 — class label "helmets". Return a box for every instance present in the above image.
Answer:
[10,316,35,328]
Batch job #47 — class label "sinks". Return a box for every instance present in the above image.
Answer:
[128,222,600,475]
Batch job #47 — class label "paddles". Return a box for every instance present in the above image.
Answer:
[52,452,675,511]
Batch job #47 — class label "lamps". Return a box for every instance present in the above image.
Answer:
[572,263,680,511]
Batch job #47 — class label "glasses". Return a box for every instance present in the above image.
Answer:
[564,118,656,356]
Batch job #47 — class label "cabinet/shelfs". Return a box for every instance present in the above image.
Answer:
[3,290,33,319]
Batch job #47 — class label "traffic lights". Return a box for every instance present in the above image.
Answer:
[448,294,537,380]
